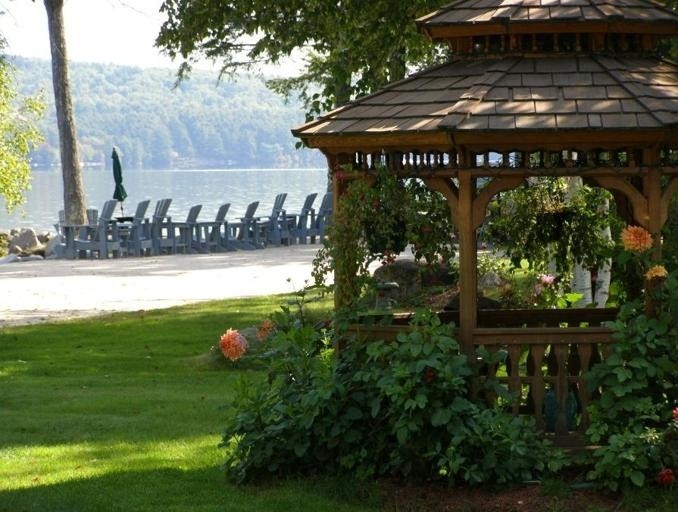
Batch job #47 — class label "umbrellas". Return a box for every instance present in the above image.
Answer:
[112,147,128,217]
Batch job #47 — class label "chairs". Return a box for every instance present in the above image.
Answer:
[55,192,334,259]
